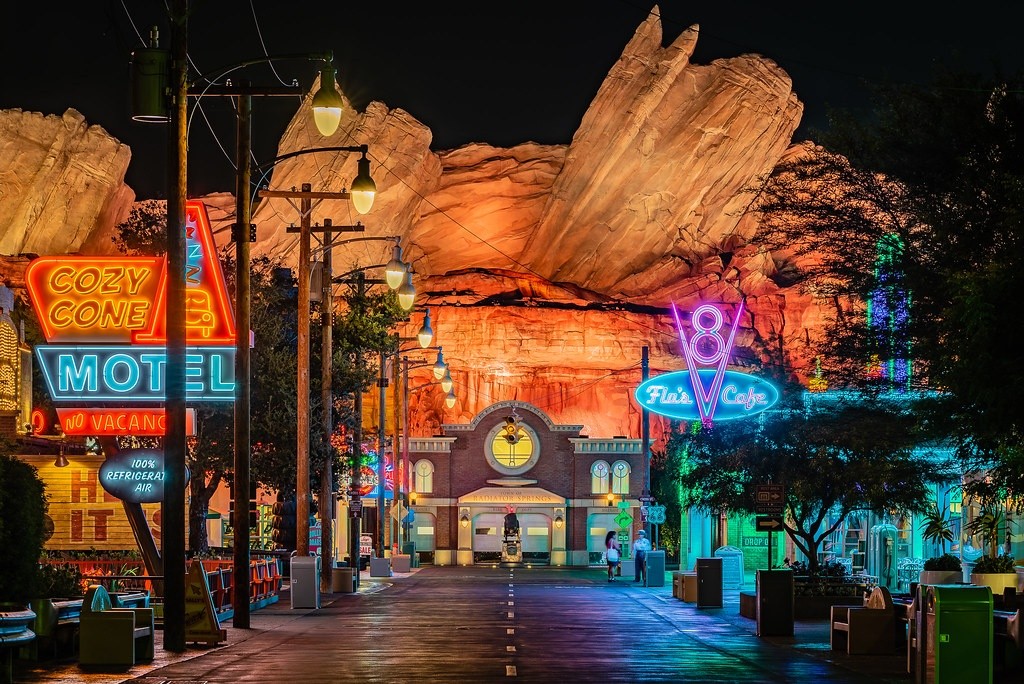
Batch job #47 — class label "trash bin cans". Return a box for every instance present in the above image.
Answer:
[332,567,357,593]
[415,553,421,567]
[290,555,323,610]
[918,584,993,684]
[696,557,724,607]
[756,568,794,638]
[644,548,664,588]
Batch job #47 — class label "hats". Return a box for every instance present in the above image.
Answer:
[637,531,645,535]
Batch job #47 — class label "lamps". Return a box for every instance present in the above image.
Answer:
[555,509,563,529]
[460,509,470,528]
[409,491,417,506]
[607,493,614,506]
[53,446,70,467]
[24,424,32,437]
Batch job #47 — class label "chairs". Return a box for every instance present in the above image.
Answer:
[370,549,392,577]
[392,547,411,573]
[332,567,358,594]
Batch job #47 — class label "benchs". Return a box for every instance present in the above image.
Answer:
[673,564,697,603]
[829,585,907,657]
[78,584,155,669]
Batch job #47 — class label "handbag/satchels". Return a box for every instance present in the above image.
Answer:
[607,539,619,562]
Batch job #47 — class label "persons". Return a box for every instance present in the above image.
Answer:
[605,530,623,583]
[632,530,652,585]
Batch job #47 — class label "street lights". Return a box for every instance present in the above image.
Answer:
[282,218,418,595]
[233,77,380,630]
[163,0,348,653]
[373,301,461,567]
[286,181,408,556]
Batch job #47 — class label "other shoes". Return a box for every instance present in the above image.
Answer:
[632,578,638,583]
[608,577,616,583]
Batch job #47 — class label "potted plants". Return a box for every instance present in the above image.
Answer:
[918,497,963,585]
[962,502,1020,595]
